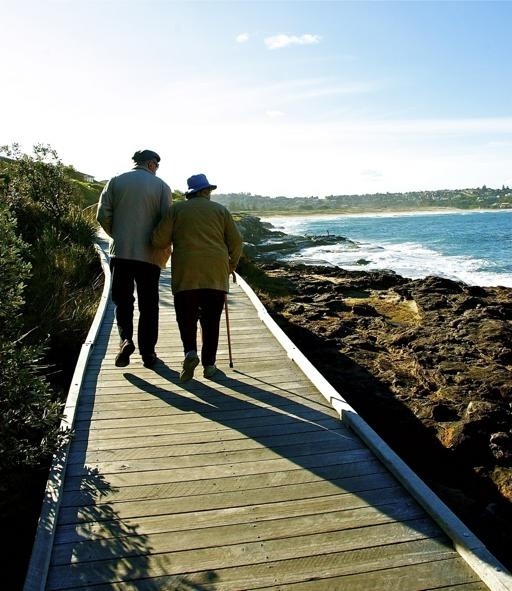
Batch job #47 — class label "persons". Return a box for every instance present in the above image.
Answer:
[150,173,245,384]
[95,150,173,370]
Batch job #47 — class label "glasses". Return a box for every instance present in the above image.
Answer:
[153,163,160,169]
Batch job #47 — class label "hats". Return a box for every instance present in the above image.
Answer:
[131,150,162,163]
[180,173,218,198]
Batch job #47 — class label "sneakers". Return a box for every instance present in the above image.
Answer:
[114,338,136,368]
[179,352,201,384]
[202,364,218,379]
[142,352,159,366]
